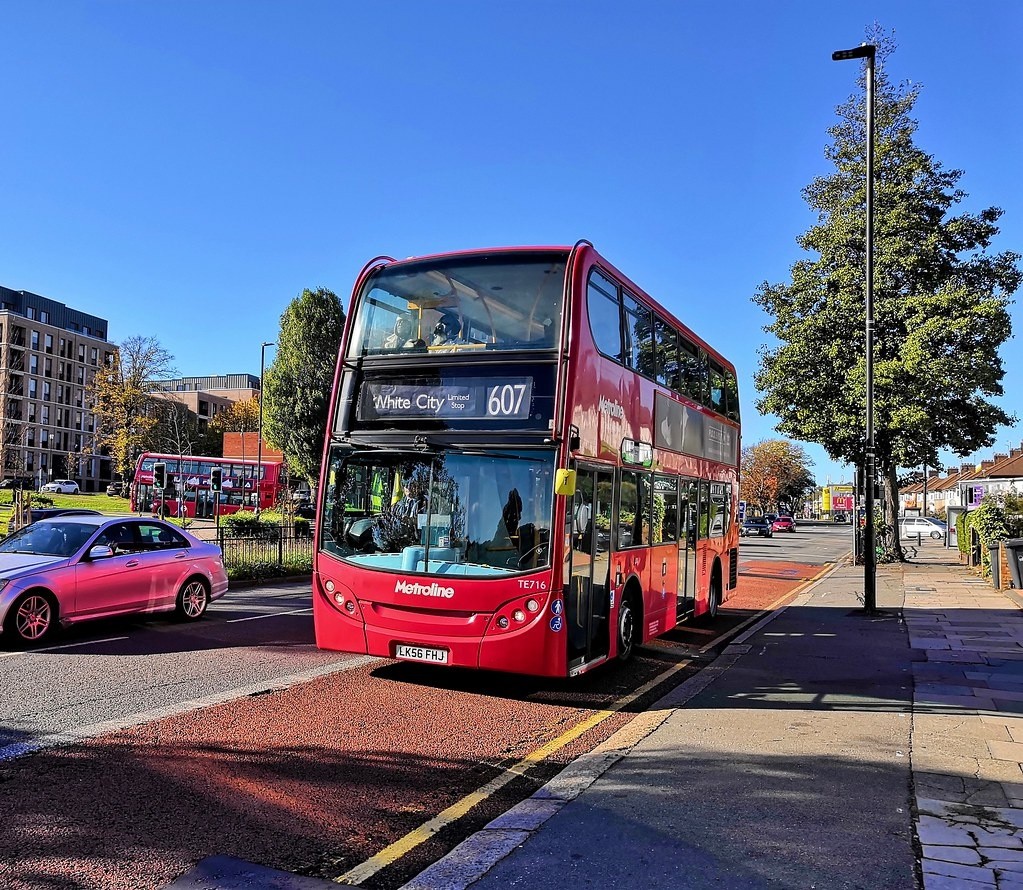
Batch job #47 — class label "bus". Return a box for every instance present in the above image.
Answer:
[313,238,742,677]
[129,451,287,519]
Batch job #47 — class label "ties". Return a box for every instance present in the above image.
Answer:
[406,499,413,517]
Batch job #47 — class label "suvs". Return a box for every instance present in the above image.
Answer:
[294,489,310,505]
[897,516,947,539]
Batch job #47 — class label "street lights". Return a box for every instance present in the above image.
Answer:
[255,342,276,523]
[49,434,54,483]
[831,40,886,618]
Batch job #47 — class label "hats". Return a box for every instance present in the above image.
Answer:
[394,313,415,337]
[434,314,462,335]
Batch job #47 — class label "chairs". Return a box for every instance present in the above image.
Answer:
[158,532,173,545]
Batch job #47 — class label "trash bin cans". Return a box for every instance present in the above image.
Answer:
[987,542,999,589]
[1004,537,1023,589]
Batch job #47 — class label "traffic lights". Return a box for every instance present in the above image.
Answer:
[154,463,174,491]
[210,467,222,492]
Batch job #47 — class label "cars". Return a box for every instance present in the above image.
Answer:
[105,482,122,497]
[41,479,80,495]
[597,524,634,550]
[0,480,31,489]
[739,513,796,537]
[833,515,845,523]
[0,507,229,646]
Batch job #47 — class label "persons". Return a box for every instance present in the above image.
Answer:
[385,313,415,347]
[430,314,471,352]
[385,470,428,545]
[53,528,81,554]
